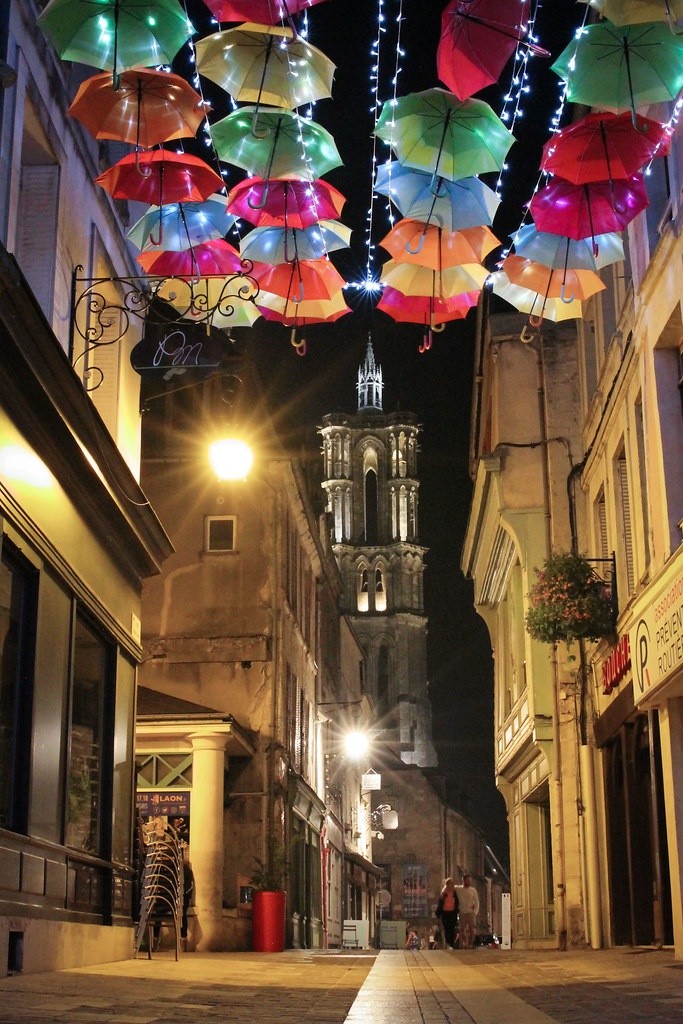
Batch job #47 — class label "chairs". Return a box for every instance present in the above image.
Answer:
[342,925,359,951]
[132,821,186,963]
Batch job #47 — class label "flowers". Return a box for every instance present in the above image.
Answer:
[528,568,618,623]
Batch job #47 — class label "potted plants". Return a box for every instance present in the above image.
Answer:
[239,822,305,953]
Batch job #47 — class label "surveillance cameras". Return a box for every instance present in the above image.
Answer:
[377,833,384,841]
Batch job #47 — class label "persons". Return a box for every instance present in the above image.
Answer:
[405,931,419,951]
[431,925,442,948]
[434,878,459,950]
[441,874,479,949]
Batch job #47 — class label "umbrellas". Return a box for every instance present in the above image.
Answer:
[37,0,353,354]
[371,0,683,354]
